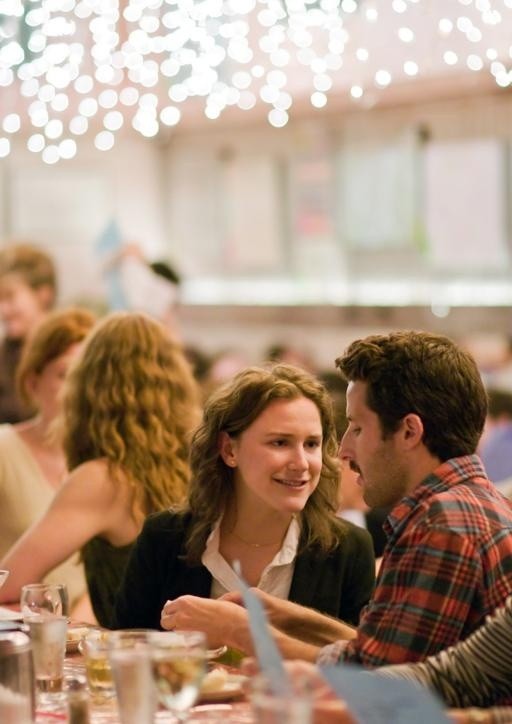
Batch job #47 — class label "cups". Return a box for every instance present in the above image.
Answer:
[103,637,157,724]
[79,638,126,705]
[20,583,65,624]
[148,627,206,723]
[20,614,70,691]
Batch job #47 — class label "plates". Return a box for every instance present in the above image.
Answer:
[67,625,103,652]
[203,672,255,705]
